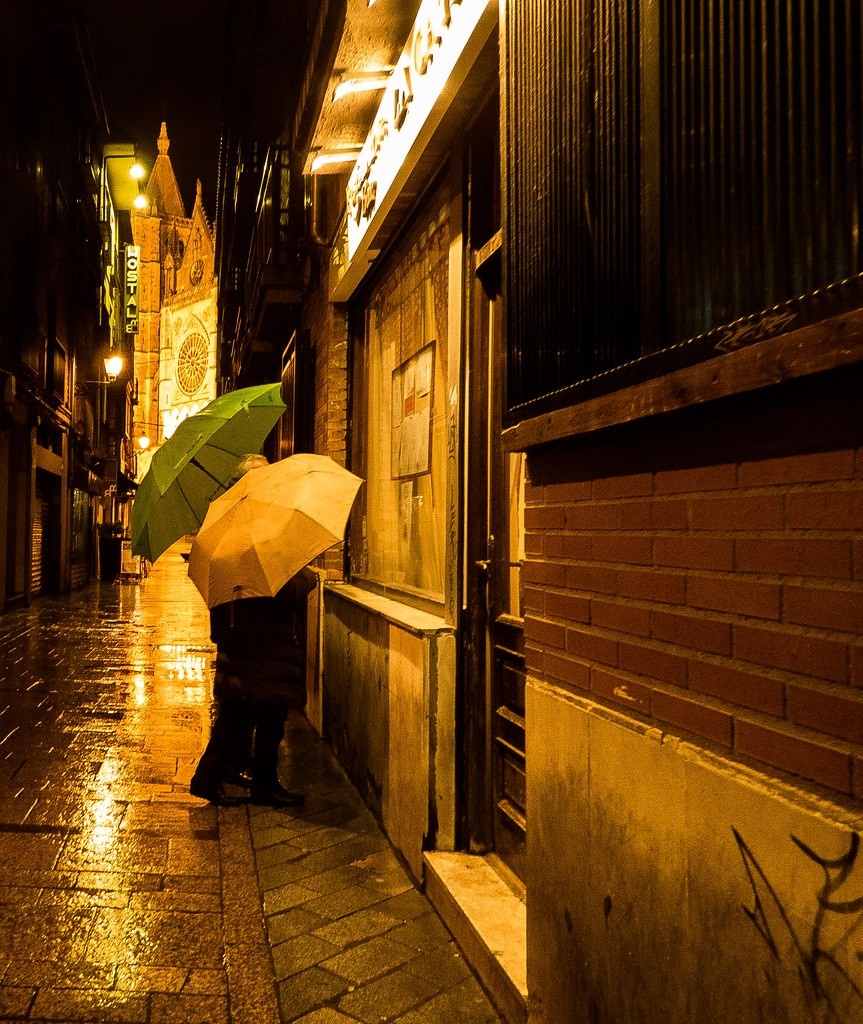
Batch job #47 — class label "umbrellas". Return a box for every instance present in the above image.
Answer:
[188,454,368,610]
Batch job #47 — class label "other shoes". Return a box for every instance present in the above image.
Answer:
[224,763,253,787]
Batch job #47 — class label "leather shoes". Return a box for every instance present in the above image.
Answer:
[252,780,305,807]
[190,774,240,807]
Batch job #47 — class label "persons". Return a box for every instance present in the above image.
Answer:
[130,383,290,565]
[226,451,278,786]
[190,564,306,809]
